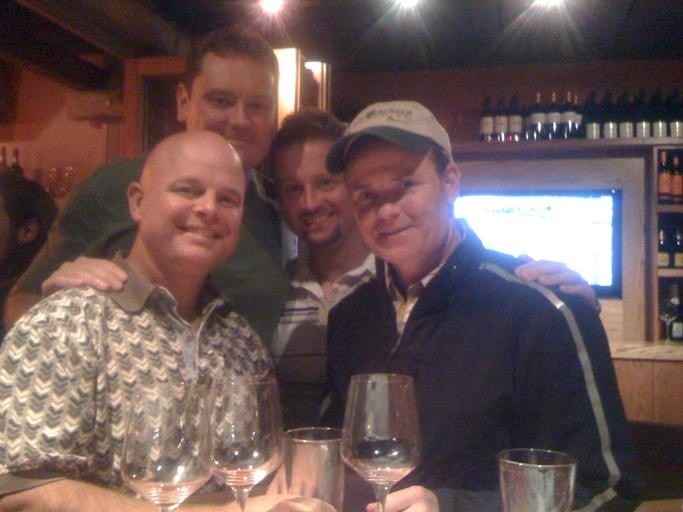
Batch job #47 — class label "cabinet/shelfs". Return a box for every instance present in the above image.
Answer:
[645,145,682,344]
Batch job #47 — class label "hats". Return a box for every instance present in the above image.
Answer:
[325,99,453,174]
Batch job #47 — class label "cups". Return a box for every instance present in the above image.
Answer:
[284,425,351,512]
[495,448,578,512]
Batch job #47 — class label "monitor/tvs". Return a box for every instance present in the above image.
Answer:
[448,189,623,300]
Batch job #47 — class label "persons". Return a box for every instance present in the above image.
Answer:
[263,108,600,437]
[2,22,295,349]
[315,99,645,512]
[0,168,60,338]
[0,127,340,512]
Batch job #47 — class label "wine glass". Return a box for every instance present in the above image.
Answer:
[197,376,284,512]
[121,371,218,512]
[339,373,423,512]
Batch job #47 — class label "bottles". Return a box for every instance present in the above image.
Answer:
[0,146,76,197]
[658,150,683,341]
[469,88,683,142]
